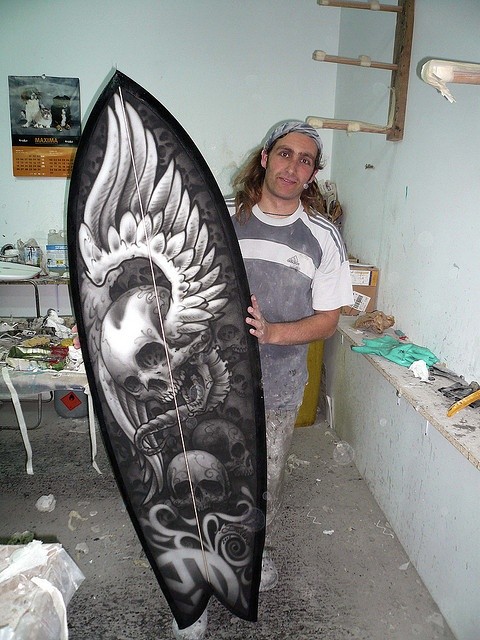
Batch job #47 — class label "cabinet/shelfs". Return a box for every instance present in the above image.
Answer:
[0,274,86,431]
[306,0,416,141]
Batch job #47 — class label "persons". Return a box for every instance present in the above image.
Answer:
[170,119,355,639]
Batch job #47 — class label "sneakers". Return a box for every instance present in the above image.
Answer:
[260,541,279,592]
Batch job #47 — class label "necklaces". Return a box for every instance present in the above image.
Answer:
[263,212,292,216]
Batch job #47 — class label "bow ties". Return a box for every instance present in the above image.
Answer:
[171,601,208,636]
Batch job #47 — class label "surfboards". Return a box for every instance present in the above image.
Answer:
[64,68,269,629]
